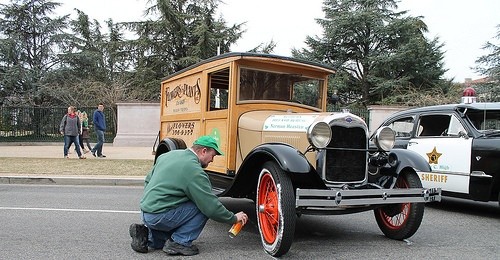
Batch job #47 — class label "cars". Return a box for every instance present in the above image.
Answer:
[368,87,500,213]
[152,52,442,258]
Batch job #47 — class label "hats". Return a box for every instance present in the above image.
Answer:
[193,135,224,158]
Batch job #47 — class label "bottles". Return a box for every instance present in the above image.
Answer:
[215,89,221,108]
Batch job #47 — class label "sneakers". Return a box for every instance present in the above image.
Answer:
[128,223,149,254]
[162,237,200,256]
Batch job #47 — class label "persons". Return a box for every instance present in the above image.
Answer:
[74,111,93,154]
[91,103,107,157]
[60,106,88,159]
[129,135,248,256]
[67,110,90,155]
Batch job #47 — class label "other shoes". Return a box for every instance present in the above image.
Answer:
[79,156,87,159]
[64,155,70,159]
[90,150,106,157]
